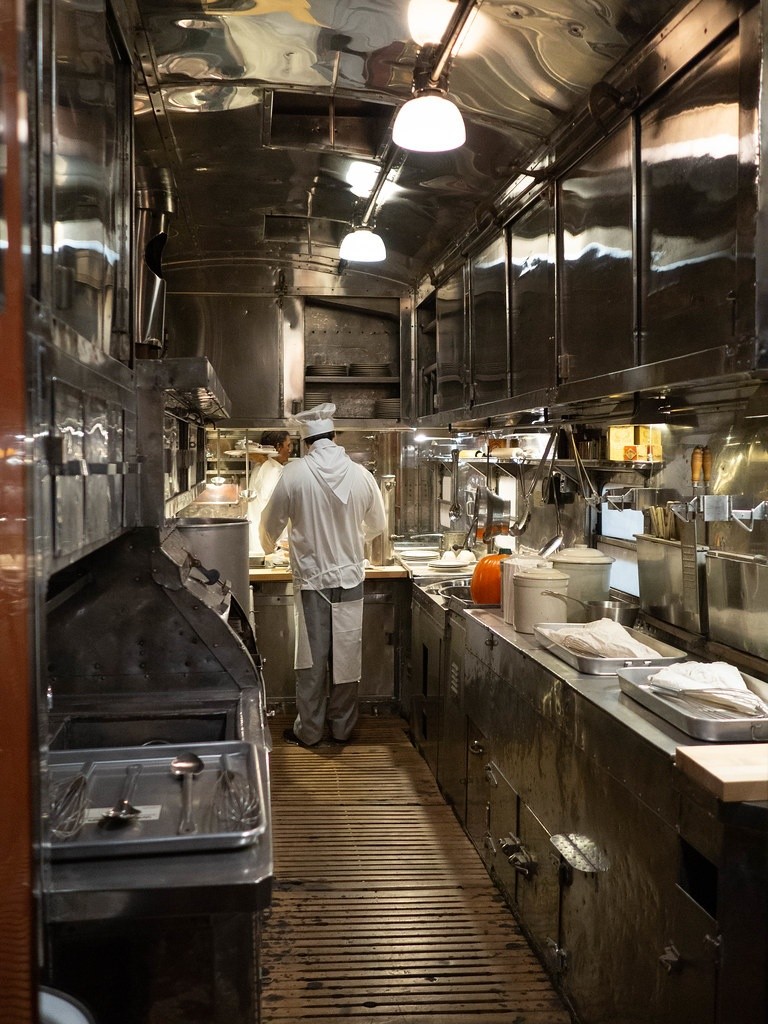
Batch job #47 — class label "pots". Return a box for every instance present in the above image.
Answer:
[546,591,642,628]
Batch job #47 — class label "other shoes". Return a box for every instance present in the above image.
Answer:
[283,727,320,749]
[332,736,347,745]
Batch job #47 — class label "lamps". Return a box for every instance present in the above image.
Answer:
[338,216,388,263]
[391,60,467,155]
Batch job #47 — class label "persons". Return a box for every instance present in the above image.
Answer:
[257,403,390,748]
[247,431,293,553]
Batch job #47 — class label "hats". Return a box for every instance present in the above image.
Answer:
[290,403,336,441]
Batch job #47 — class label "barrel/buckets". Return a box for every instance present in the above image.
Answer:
[361,473,396,567]
[178,517,251,619]
[548,543,617,622]
[501,550,554,625]
[511,565,570,637]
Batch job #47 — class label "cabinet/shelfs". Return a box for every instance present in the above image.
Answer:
[304,376,401,432]
[411,0,768,430]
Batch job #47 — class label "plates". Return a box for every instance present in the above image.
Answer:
[399,551,440,561]
[427,560,469,571]
[348,363,391,377]
[375,398,402,419]
[307,364,348,376]
[305,392,332,410]
